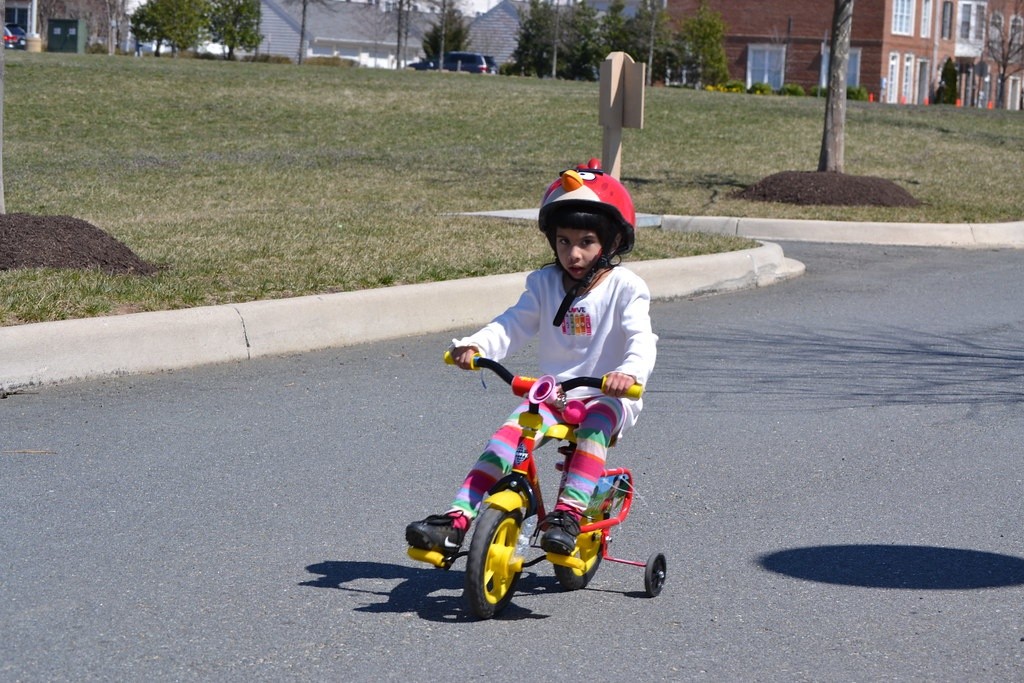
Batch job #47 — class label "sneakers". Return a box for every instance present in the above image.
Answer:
[406,510,466,555]
[534,511,582,556]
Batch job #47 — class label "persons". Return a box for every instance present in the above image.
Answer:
[405,158,659,570]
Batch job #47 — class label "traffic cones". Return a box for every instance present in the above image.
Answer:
[955,99,960,106]
[867,91,874,102]
[924,97,929,106]
[987,101,992,109]
[901,95,907,105]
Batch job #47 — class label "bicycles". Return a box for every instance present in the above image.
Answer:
[406,349,667,622]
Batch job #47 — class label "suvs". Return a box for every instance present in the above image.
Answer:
[407,51,499,74]
[4,23,28,51]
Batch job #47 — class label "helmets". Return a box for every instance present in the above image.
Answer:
[538,158,636,251]
